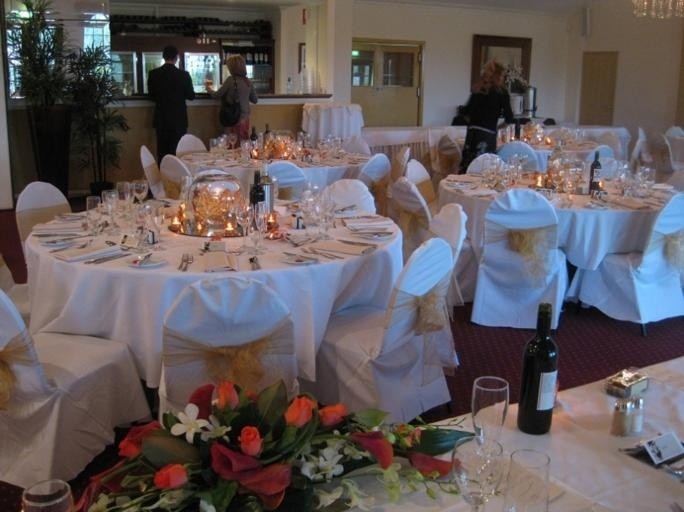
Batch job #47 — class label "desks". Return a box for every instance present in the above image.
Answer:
[429,172,681,299]
[355,124,465,173]
[24,198,406,431]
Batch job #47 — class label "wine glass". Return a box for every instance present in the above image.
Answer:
[299,179,339,245]
[89,174,162,258]
[236,199,270,258]
[479,151,659,207]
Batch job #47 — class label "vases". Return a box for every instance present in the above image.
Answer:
[507,93,523,115]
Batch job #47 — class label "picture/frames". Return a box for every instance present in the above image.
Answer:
[297,43,306,74]
[471,34,531,95]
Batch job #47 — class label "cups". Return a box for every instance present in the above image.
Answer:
[470,376,510,455]
[207,123,343,164]
[497,122,585,148]
[23,479,76,512]
[449,436,503,512]
[504,447,552,511]
[203,79,213,91]
[179,175,198,200]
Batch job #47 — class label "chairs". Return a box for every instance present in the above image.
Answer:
[406,158,433,227]
[1,288,154,494]
[3,260,34,330]
[156,272,297,436]
[574,193,682,339]
[471,187,571,333]
[267,160,307,204]
[17,177,73,247]
[513,120,683,173]
[135,139,163,199]
[157,150,197,201]
[392,177,479,304]
[392,145,413,182]
[422,200,470,366]
[320,177,379,218]
[315,234,454,423]
[175,134,209,157]
[356,152,391,220]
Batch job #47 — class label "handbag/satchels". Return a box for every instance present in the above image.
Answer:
[219,102,241,127]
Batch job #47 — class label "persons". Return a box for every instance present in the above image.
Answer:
[452,105,467,125]
[205,53,258,149]
[459,57,514,175]
[148,44,195,169]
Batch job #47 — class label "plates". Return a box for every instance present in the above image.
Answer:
[55,215,87,225]
[39,235,74,247]
[279,254,318,266]
[364,230,395,241]
[130,257,167,268]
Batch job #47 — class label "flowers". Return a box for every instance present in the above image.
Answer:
[76,370,476,512]
[502,61,530,93]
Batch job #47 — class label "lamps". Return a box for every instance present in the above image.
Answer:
[631,0,684,21]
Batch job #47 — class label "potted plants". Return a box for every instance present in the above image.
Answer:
[3,0,116,197]
[63,69,131,197]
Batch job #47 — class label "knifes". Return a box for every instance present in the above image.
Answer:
[284,252,316,262]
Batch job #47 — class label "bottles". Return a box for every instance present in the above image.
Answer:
[247,156,273,205]
[611,399,631,438]
[289,214,297,230]
[629,394,645,434]
[247,68,272,94]
[223,47,270,66]
[110,15,267,36]
[516,302,560,438]
[121,80,133,97]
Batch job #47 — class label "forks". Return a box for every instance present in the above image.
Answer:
[176,254,194,272]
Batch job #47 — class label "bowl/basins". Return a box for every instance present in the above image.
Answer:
[170,173,257,238]
